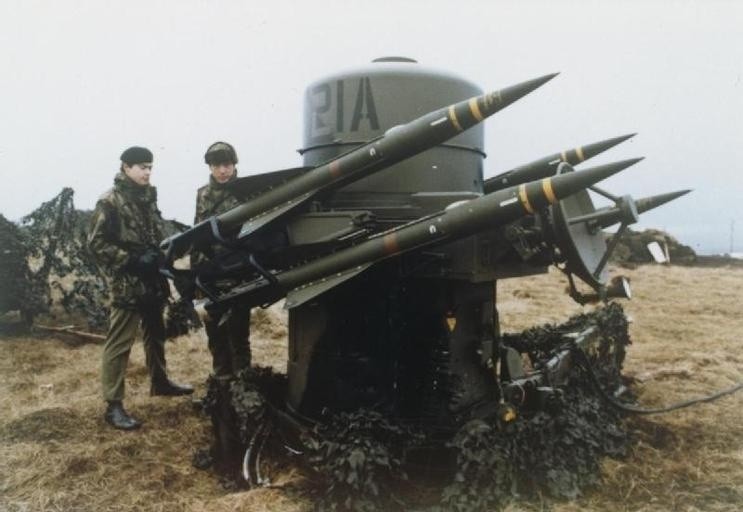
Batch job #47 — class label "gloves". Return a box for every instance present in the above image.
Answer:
[136,252,164,271]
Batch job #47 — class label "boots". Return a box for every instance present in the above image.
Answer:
[105,399,141,430]
[150,374,195,396]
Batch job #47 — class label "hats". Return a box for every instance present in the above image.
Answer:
[205,142,238,164]
[120,147,153,164]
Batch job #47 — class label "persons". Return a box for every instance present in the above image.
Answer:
[189,142,251,374]
[87,146,195,431]
